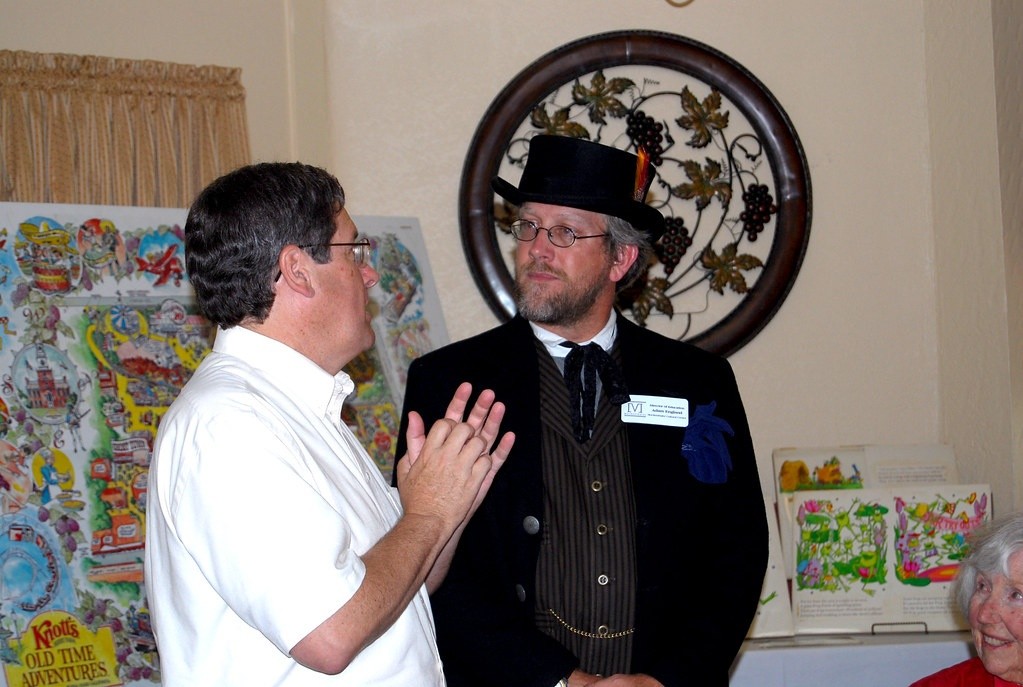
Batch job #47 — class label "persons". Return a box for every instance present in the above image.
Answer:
[391,134,770,687]
[908,513,1023,687]
[142,160,513,687]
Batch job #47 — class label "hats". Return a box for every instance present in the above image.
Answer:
[489,134,666,245]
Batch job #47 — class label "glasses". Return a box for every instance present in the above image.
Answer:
[507,218,610,248]
[274,237,370,283]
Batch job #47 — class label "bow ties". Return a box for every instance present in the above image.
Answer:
[559,340,631,431]
